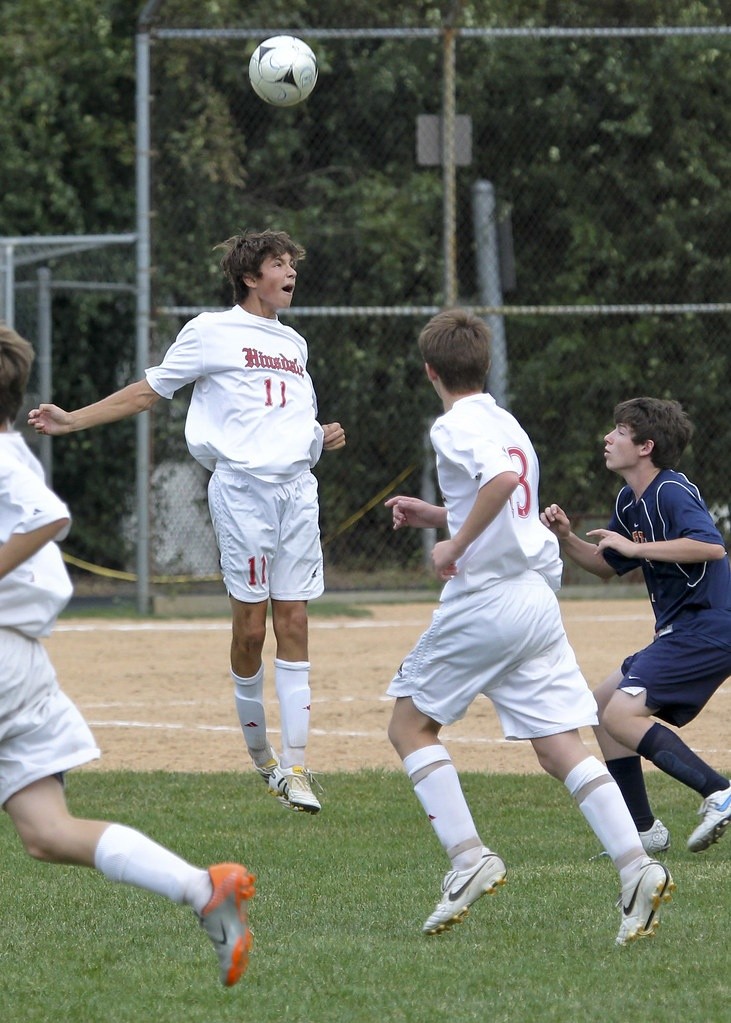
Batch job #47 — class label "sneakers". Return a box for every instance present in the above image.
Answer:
[253,745,282,783]
[421,846,507,936]
[687,780,731,853]
[637,819,672,856]
[613,859,677,948]
[197,862,255,986]
[269,763,323,816]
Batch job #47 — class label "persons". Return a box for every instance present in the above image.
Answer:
[540,398,731,861]
[385,309,676,946]
[27,229,346,815]
[0,322,254,986]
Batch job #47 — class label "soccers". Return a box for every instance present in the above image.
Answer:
[247,36,319,107]
